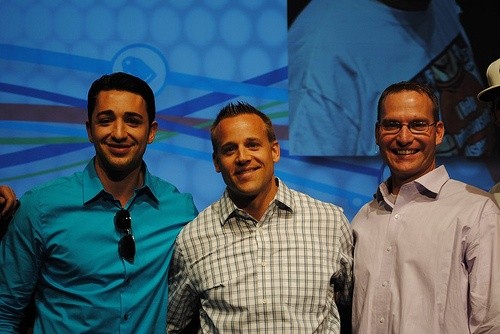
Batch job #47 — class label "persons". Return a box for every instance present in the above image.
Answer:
[350,81,500,334]
[1,72,197,334]
[479,60,500,205]
[0,186,17,217]
[165,102,354,334]
[287,0,493,156]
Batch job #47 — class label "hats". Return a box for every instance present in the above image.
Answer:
[476,58,500,103]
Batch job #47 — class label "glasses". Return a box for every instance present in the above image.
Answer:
[113,208,136,264]
[378,118,435,135]
